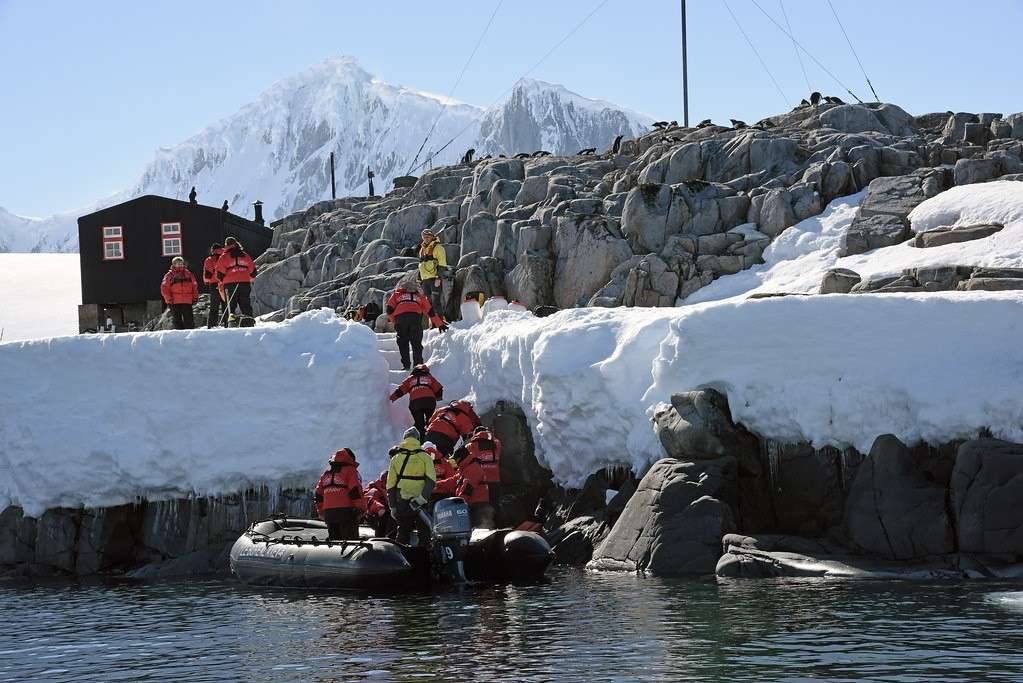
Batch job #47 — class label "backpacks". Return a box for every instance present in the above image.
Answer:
[400,282,417,293]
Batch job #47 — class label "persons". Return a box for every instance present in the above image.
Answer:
[386,281,449,369]
[314,448,367,539]
[387,426,436,544]
[343,300,380,329]
[364,470,397,540]
[161,257,198,329]
[390,364,443,443]
[417,229,446,330]
[466,426,504,529]
[426,399,481,458]
[434,447,489,528]
[204,243,229,329]
[214,237,258,327]
[421,441,455,514]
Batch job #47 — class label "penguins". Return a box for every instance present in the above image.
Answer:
[801,92,846,106]
[461,147,599,162]
[695,119,716,128]
[612,134,624,154]
[728,118,776,129]
[651,121,677,131]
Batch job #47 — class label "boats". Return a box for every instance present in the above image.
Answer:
[230,511,559,597]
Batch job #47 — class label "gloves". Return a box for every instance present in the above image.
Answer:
[167,302,173,308]
[193,300,198,305]
[409,497,425,511]
[378,508,385,517]
[437,397,442,401]
[439,325,450,334]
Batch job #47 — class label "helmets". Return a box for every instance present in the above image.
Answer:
[380,470,388,481]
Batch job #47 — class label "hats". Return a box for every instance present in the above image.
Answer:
[212,244,222,250]
[449,453,454,459]
[473,426,487,436]
[404,427,420,438]
[389,446,400,459]
[421,441,437,450]
[225,237,236,245]
[345,448,355,457]
[454,447,469,457]
[414,364,430,372]
[421,228,434,235]
